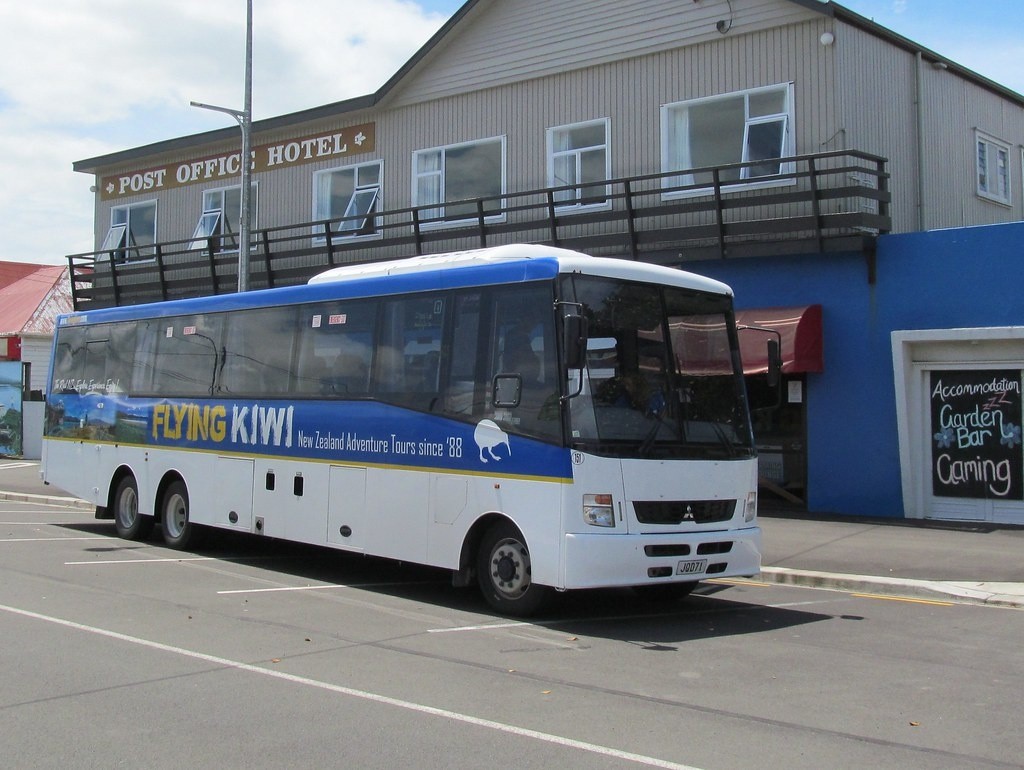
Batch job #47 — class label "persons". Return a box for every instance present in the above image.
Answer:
[502,338,549,433]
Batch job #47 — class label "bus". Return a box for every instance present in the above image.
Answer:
[40,240,784,618]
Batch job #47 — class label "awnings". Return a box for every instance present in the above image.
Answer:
[637,303,825,374]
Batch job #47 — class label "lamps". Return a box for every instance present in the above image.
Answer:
[821,32,834,47]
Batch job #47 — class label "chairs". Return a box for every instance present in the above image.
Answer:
[502,328,548,409]
